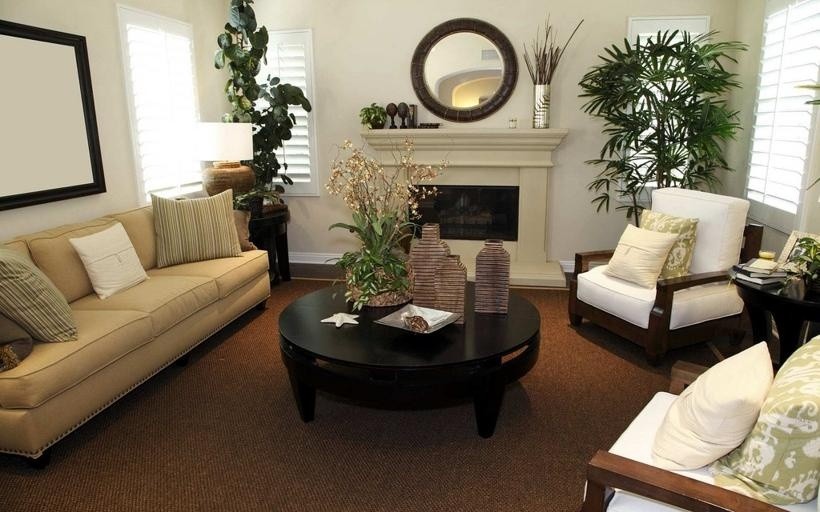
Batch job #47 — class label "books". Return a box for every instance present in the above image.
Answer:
[728,257,790,291]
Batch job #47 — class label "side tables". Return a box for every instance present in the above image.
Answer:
[245,211,291,287]
[728,261,820,369]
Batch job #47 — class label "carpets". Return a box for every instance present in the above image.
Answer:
[3,277,718,511]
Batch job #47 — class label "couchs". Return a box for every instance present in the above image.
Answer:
[579,359,820,512]
[569,186,765,365]
[0,195,271,471]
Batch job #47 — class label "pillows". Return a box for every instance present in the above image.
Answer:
[635,209,699,290]
[604,223,678,290]
[0,246,79,342]
[70,222,151,301]
[711,330,820,504]
[225,209,257,250]
[150,188,244,268]
[654,341,773,471]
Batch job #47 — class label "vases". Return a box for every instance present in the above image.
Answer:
[533,85,550,131]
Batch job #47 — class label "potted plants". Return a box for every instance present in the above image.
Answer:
[214,0,312,218]
[792,237,819,300]
[361,103,386,129]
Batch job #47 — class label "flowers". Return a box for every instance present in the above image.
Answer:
[324,132,449,311]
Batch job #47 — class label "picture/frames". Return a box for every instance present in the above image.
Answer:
[0,19,107,215]
[777,230,820,270]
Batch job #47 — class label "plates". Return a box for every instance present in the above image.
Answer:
[375,303,461,335]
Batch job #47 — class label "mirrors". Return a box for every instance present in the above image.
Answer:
[410,17,519,124]
